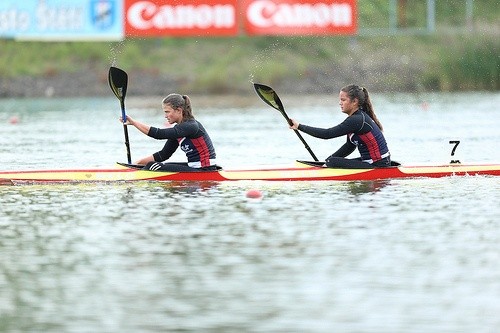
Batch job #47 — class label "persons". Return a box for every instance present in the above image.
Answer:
[286,80,394,170]
[115,93,221,176]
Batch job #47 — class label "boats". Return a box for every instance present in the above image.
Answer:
[1,153,500,184]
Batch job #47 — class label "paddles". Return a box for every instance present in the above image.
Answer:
[254,83,320,161]
[108,66,133,164]
